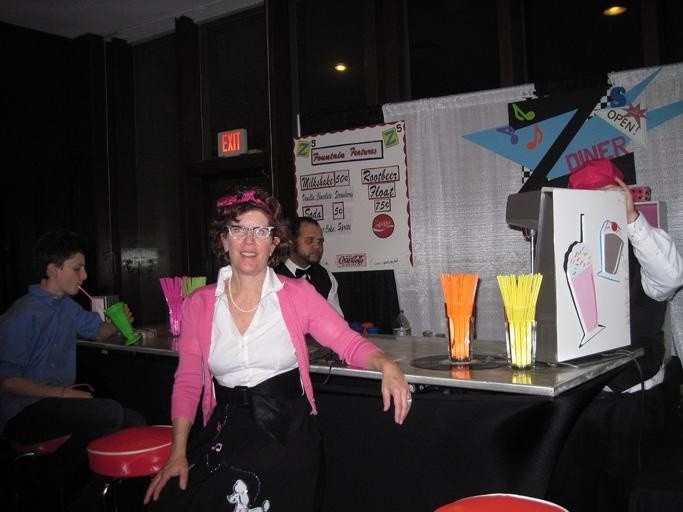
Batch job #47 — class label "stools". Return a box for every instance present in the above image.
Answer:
[87,425,174,512]
[433,493,568,512]
[10,434,72,512]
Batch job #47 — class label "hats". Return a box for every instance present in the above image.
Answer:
[568,158,624,189]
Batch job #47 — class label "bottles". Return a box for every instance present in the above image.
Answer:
[392,310,411,337]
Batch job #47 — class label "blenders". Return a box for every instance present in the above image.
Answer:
[506,186,631,368]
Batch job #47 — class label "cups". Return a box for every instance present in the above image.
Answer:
[446,315,475,364]
[504,320,537,370]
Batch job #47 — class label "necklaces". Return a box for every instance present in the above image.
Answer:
[227,276,264,313]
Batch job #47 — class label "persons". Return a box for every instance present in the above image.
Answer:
[565,156,681,402]
[142,170,416,511]
[0,234,150,511]
[272,214,345,319]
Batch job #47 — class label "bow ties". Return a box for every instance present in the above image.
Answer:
[295,267,312,279]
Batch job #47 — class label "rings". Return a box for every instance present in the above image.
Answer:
[407,399,413,403]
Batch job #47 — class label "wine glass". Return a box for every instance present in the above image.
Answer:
[100,302,141,347]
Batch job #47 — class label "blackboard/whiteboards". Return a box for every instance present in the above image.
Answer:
[291,120,414,274]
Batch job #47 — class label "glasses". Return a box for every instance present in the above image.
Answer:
[226,225,275,239]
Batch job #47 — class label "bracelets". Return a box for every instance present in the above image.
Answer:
[61,386,66,398]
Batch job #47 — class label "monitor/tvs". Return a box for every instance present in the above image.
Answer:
[331,269,402,334]
